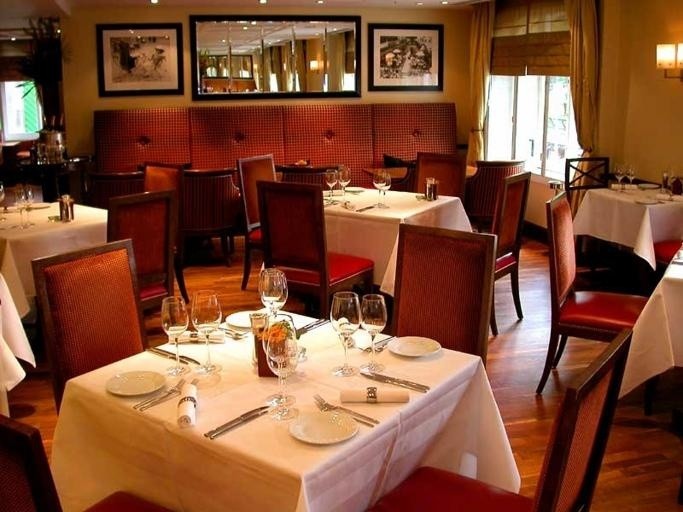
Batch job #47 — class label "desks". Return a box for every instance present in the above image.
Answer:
[617,247,682,399]
[48,307,520,511]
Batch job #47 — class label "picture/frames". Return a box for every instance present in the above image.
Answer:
[95,22,186,99]
[366,20,445,93]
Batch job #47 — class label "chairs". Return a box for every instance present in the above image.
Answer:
[32,143,533,415]
[376,328,632,512]
[564,156,683,265]
[534,192,654,401]
[0,413,166,510]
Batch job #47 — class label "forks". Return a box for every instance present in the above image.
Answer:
[358,335,396,353]
[315,400,375,426]
[313,394,379,424]
[222,328,248,341]
[133,378,186,409]
[373,337,395,353]
[218,327,248,336]
[141,377,199,411]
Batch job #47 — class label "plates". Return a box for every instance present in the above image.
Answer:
[387,335,442,358]
[225,310,258,330]
[288,412,361,445]
[108,369,167,397]
[28,202,51,211]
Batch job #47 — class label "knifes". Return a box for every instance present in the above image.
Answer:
[361,374,428,394]
[203,405,272,437]
[209,410,269,439]
[154,346,200,365]
[373,373,429,390]
[147,348,188,366]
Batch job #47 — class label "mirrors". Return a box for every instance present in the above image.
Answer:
[188,14,361,102]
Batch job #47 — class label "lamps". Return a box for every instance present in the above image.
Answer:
[653,42,682,83]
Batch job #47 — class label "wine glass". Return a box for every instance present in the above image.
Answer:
[0,183,34,231]
[258,268,289,312]
[610,164,682,204]
[318,164,393,213]
[162,296,190,376]
[262,314,298,406]
[191,290,223,376]
[266,330,299,421]
[360,294,386,374]
[329,292,361,377]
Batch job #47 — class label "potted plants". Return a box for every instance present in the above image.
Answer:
[9,13,68,164]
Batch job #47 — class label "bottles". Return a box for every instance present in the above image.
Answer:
[40,112,67,132]
[425,177,440,203]
[28,132,69,165]
[57,193,75,221]
